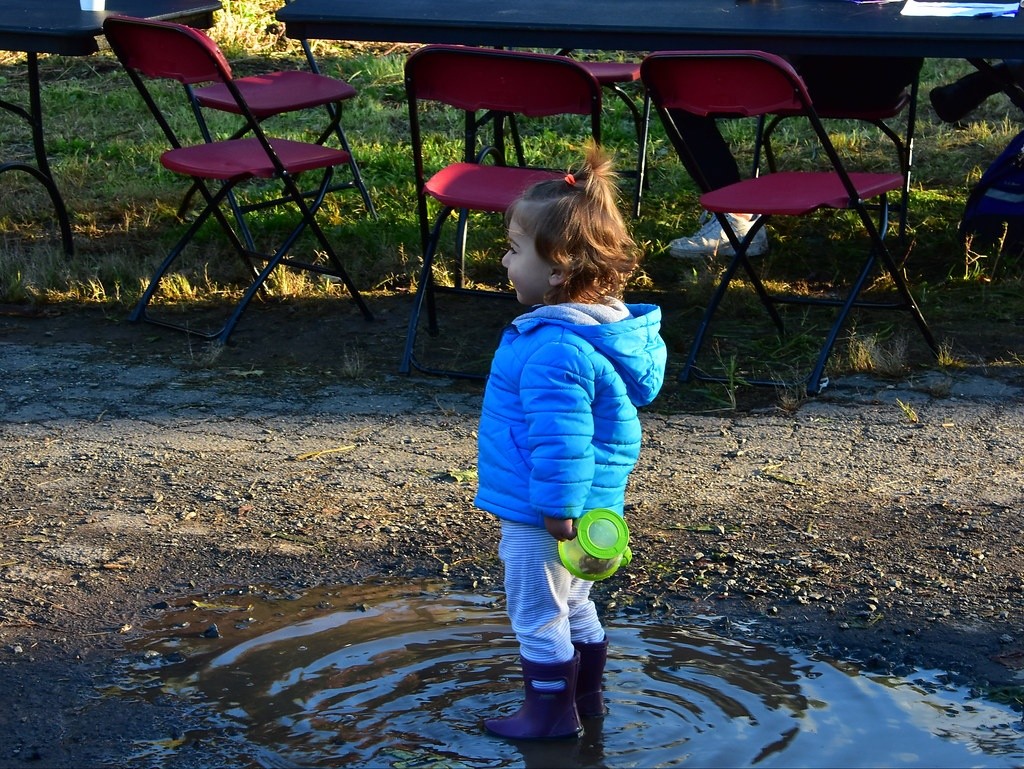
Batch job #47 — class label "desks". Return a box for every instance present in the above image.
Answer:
[0,0,224,261]
[274,1,1024,296]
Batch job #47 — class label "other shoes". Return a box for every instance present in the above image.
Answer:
[931,72,993,122]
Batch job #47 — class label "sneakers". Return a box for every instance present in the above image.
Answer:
[669,213,768,260]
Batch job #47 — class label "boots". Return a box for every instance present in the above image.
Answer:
[571,633,609,719]
[484,649,585,740]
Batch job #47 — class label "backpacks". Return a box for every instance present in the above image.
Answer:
[957,130,1024,245]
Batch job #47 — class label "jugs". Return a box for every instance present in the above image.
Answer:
[557,508,633,582]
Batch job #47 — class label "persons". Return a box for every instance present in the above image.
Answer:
[930,60,1024,123]
[656,56,923,258]
[474,136,666,744]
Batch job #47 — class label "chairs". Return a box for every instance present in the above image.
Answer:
[99,13,377,350]
[181,36,375,255]
[395,37,659,381]
[636,51,942,397]
